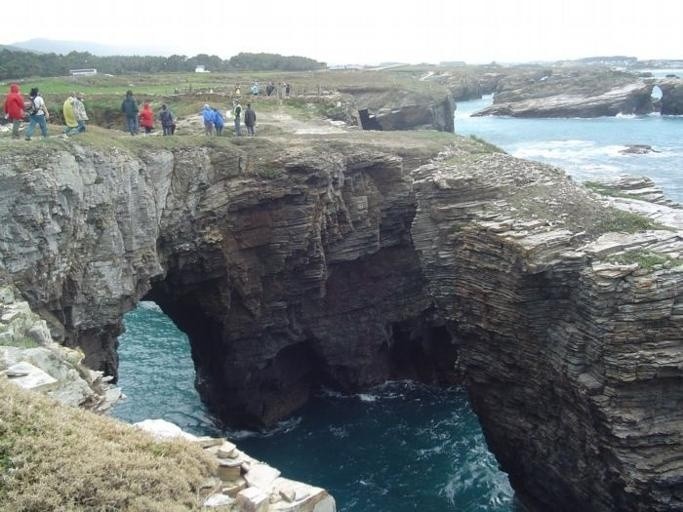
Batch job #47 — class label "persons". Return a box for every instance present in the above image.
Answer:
[244,103,257,136]
[120,89,141,136]
[234,81,291,101]
[212,108,225,135]
[159,105,172,135]
[62,91,78,132]
[4,83,30,138]
[203,104,215,135]
[70,94,89,134]
[25,87,49,141]
[232,99,243,136]
[139,103,154,133]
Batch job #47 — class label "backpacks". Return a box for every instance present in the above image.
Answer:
[26,96,39,114]
